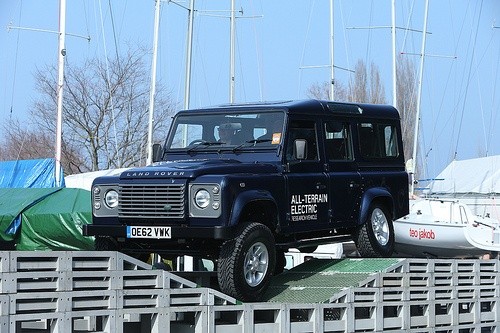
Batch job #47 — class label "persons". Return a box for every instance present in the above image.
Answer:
[215,123,246,146]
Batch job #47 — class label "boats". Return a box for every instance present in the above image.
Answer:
[376,155,500,262]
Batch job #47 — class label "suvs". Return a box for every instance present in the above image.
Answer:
[82,99,409,305]
